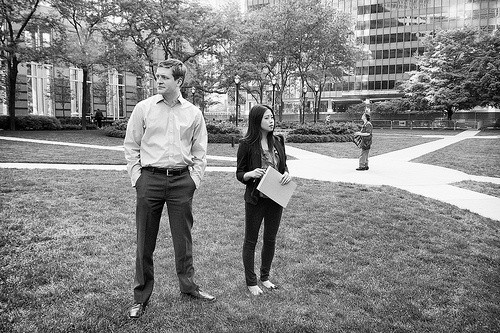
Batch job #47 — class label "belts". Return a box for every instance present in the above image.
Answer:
[143,166,188,176]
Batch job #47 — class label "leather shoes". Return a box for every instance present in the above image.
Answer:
[181,289,217,302]
[129,304,144,318]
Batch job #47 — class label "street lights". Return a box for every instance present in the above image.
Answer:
[235,74,241,127]
[191,86,195,104]
[302,87,307,123]
[314,87,318,123]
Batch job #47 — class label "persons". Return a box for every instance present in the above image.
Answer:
[94,109,104,129]
[354,114,373,170]
[123,59,215,319]
[237,104,292,295]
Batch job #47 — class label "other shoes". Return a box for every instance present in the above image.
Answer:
[356,166,369,171]
[247,285,262,295]
[259,280,278,289]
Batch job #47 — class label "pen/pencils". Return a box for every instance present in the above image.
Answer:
[253,168,267,182]
